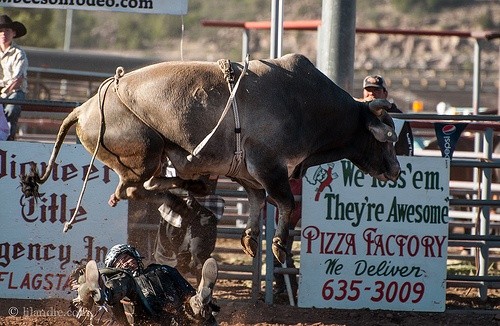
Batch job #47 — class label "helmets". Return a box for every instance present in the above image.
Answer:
[104,242,141,269]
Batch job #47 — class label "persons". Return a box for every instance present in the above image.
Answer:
[363,74,415,157]
[107,157,225,287]
[0,13,29,141]
[84,241,220,326]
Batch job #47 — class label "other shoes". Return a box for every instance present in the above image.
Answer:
[195,257,219,307]
[85,259,113,308]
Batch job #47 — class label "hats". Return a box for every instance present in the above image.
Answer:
[0,14,28,39]
[363,75,390,91]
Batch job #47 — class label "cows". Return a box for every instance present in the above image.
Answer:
[17,53,403,269]
